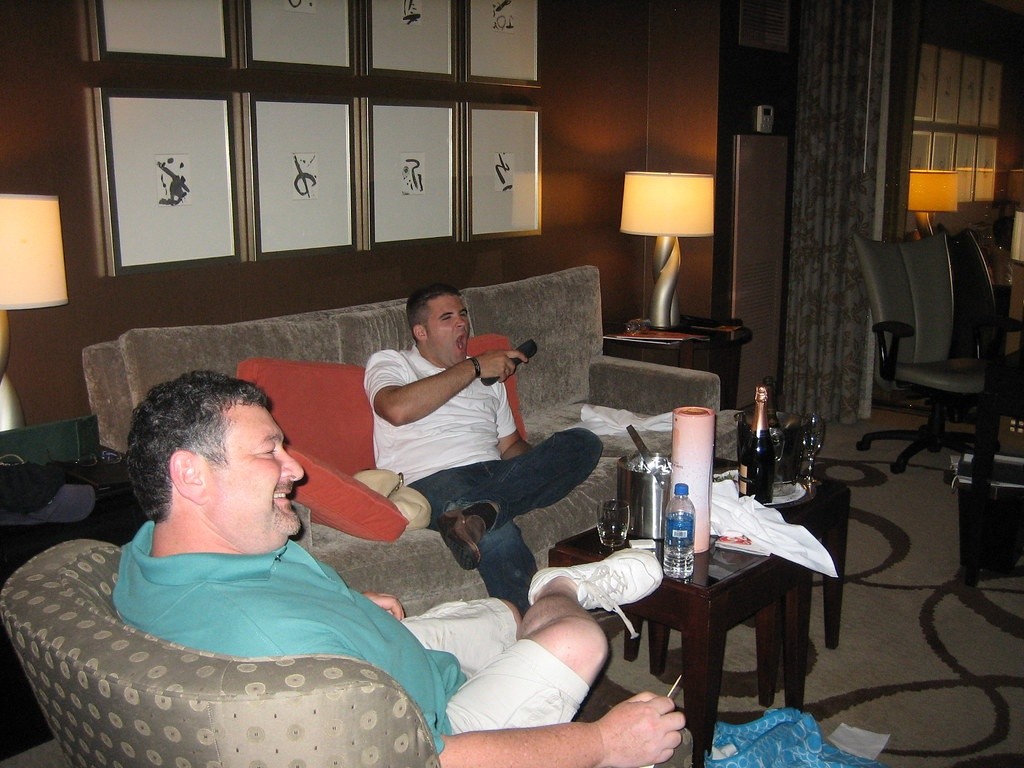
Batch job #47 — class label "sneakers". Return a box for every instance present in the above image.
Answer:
[528,548,663,639]
[437,510,486,570]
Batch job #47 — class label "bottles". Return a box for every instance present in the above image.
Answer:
[663,483,696,578]
[762,375,781,429]
[738,385,775,504]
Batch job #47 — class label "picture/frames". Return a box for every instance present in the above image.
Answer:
[914,43,1004,131]
[85,1,544,89]
[93,87,544,278]
[910,131,998,203]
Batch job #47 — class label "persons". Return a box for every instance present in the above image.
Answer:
[364,283,603,620]
[113,370,686,768]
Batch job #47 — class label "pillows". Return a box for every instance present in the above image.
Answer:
[282,445,410,542]
[466,333,526,441]
[238,358,376,475]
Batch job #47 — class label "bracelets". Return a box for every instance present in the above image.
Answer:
[467,357,481,378]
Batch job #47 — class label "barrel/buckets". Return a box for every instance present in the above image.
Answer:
[617,424,670,539]
[735,410,805,495]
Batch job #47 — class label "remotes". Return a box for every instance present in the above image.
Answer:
[481,340,539,386]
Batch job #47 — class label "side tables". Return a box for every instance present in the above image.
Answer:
[1,501,140,761]
[604,327,753,411]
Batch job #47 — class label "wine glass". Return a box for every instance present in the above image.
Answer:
[801,413,824,486]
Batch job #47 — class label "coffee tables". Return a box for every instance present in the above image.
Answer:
[548,455,850,768]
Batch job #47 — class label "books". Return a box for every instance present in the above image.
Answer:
[714,536,771,557]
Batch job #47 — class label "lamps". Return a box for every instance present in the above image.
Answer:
[620,171,715,330]
[1009,209,1024,265]
[908,168,958,236]
[1007,170,1024,202]
[0,193,69,430]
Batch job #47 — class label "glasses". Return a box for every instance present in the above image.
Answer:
[386,472,404,498]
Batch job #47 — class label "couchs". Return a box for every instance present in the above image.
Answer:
[82,266,739,618]
[1,538,695,768]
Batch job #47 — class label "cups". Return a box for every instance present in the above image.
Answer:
[596,499,630,547]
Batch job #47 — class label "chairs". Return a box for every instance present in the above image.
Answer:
[936,225,1022,359]
[851,231,1000,476]
